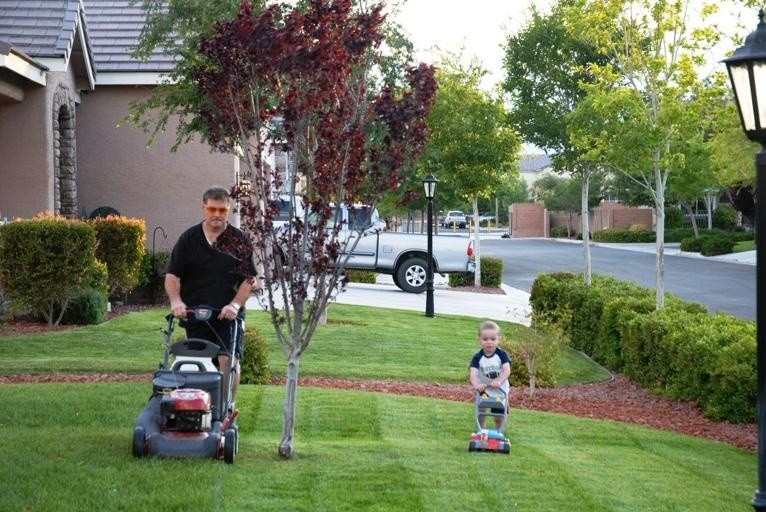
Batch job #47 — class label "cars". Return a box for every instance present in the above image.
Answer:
[432,209,500,228]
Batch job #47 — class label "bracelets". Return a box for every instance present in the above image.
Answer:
[229,301,242,312]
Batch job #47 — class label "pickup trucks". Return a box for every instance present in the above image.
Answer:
[257,206,474,294]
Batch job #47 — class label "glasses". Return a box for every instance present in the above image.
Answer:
[206,207,229,215]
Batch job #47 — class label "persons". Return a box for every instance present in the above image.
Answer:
[163,186,256,418]
[468,320,513,436]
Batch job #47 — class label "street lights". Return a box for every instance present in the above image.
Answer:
[718,9,765,511]
[422,172,439,318]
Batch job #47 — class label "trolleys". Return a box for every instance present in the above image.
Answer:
[131,305,241,464]
